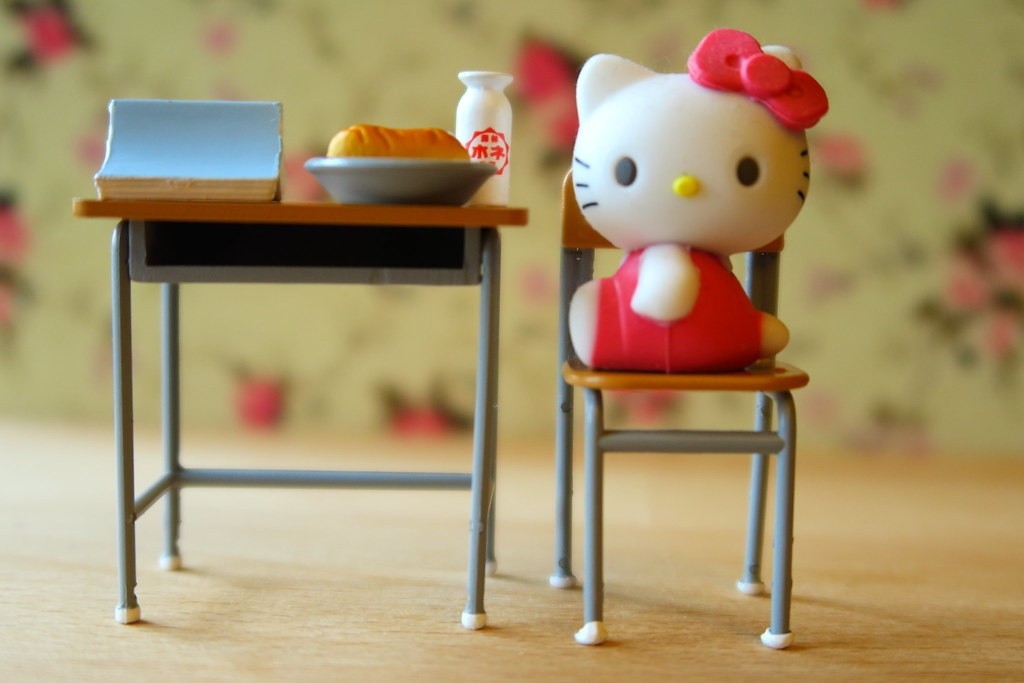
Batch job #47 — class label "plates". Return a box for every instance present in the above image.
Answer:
[303,157,497,205]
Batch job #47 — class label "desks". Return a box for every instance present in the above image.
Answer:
[73,197,528,629]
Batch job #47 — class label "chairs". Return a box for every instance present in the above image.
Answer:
[550,168,809,650]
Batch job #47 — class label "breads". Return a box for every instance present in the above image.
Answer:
[327,126,470,162]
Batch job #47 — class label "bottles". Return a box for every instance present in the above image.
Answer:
[455,70,514,204]
